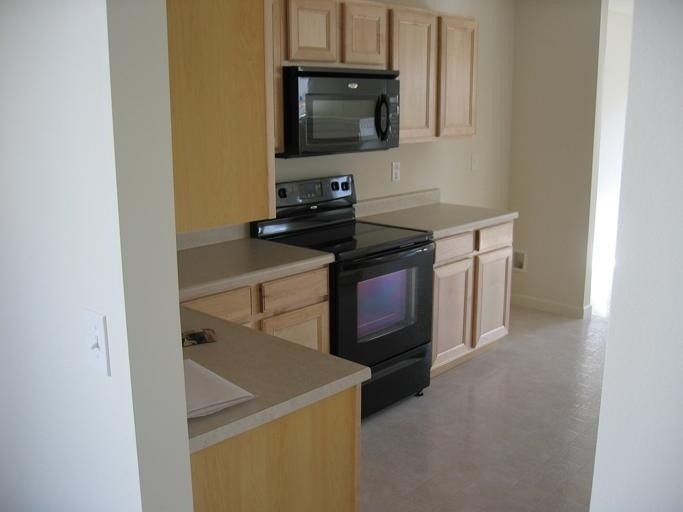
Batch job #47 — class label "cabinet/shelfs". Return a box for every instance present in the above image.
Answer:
[268,0,479,155]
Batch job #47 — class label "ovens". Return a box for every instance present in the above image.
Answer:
[327,239,434,421]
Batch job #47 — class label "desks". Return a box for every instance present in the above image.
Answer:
[172,200,519,512]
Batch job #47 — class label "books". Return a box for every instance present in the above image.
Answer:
[183,358,255,420]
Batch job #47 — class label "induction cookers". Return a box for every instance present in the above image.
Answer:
[267,218,434,267]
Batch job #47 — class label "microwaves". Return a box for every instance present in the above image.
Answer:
[280,64,402,160]
